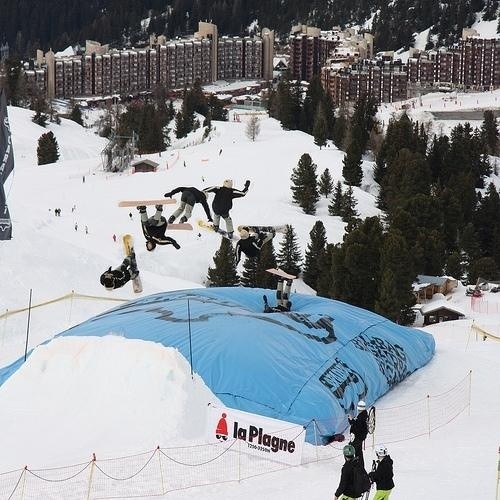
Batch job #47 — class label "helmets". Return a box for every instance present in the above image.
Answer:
[223,179,233,188]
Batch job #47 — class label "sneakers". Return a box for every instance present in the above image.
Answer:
[168,215,175,224]
[137,206,146,214]
[276,275,283,282]
[284,278,292,285]
[213,224,219,232]
[228,232,234,239]
[155,204,163,211]
[180,216,188,223]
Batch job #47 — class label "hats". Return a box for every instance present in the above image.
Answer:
[376,445,387,457]
[358,401,367,412]
[237,229,249,240]
[343,445,359,463]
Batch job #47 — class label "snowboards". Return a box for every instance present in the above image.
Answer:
[267,267,297,279]
[238,225,293,232]
[117,200,176,205]
[166,224,193,231]
[199,220,239,242]
[123,235,143,293]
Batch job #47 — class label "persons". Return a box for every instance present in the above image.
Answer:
[100,248,138,290]
[235,225,276,265]
[136,204,181,251]
[333,445,371,500]
[347,401,368,468]
[263,278,293,313]
[201,179,251,239]
[369,446,395,499]
[164,186,213,225]
